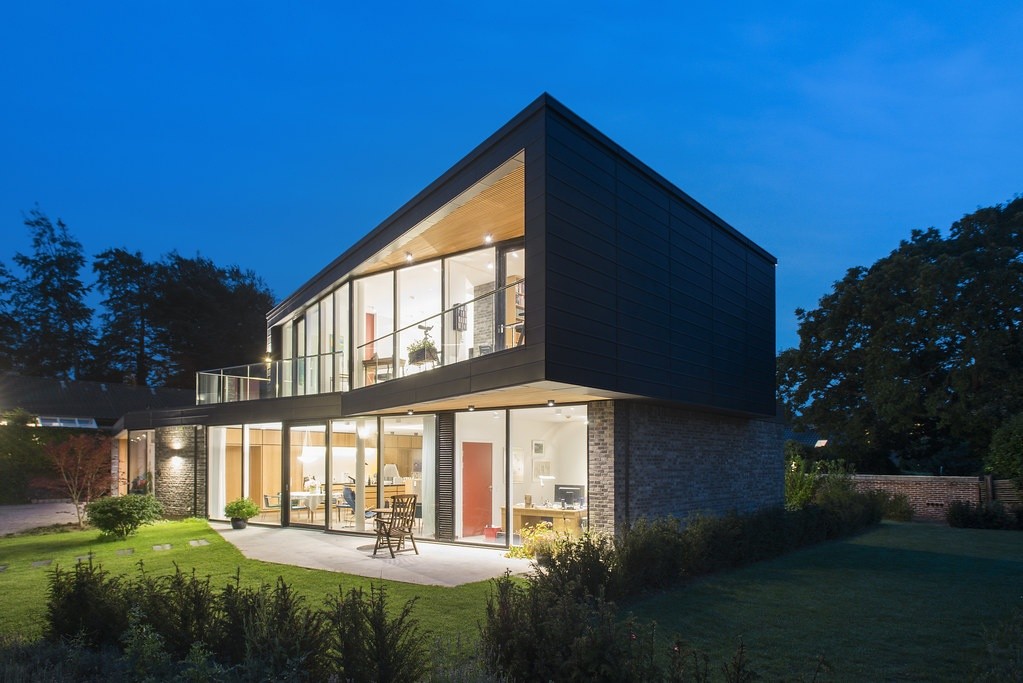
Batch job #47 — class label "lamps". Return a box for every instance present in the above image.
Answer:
[384,464,400,508]
[265,352,272,362]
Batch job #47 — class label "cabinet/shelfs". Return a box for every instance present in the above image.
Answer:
[506,275,524,348]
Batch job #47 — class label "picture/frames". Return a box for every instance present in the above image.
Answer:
[533,459,551,482]
[532,441,544,455]
[504,447,524,483]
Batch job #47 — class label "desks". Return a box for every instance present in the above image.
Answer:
[287,491,341,524]
[373,508,415,551]
[502,504,586,542]
[361,358,405,386]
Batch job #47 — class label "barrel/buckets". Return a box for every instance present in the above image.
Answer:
[484,525,500,542]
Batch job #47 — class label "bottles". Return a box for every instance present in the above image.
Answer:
[320,487,323,494]
[561,499,565,510]
[580,499,583,509]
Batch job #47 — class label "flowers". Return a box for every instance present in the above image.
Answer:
[304,479,321,487]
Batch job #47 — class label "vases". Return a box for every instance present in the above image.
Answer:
[311,485,320,494]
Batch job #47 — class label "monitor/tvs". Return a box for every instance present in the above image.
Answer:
[554,485,585,506]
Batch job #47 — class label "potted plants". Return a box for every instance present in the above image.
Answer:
[406,340,438,363]
[224,496,261,529]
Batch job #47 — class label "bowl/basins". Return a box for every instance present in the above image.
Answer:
[384,481,391,485]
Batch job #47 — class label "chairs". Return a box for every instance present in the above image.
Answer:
[264,489,420,558]
